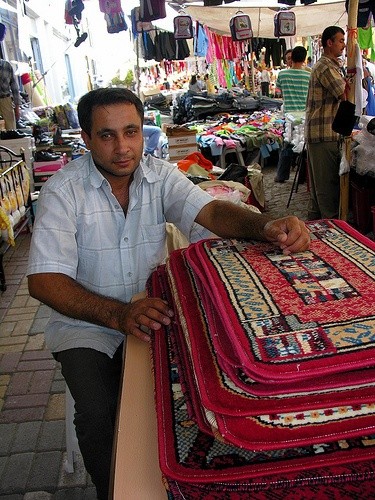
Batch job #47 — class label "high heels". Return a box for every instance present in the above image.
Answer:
[32,126,50,142]
[16,121,26,129]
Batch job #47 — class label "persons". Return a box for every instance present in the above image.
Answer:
[143,116,161,159]
[283,49,314,73]
[163,78,170,91]
[274,45,311,184]
[189,73,215,95]
[0,57,20,131]
[26,88,309,500]
[306,25,348,221]
[238,65,271,97]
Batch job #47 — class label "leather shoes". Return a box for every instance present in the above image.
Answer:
[34,150,60,162]
[0,130,26,140]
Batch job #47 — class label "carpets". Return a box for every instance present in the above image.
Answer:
[146,218,375,500]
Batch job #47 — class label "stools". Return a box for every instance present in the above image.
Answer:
[221,140,245,168]
[65,384,82,473]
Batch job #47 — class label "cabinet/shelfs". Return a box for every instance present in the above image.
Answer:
[0,137,35,192]
[33,152,66,192]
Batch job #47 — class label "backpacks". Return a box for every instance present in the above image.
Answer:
[104,8,127,33]
[229,11,253,41]
[274,6,296,37]
[194,20,209,57]
[65,0,83,24]
[139,0,166,21]
[131,7,152,32]
[173,10,194,39]
[99,0,121,16]
[140,31,190,62]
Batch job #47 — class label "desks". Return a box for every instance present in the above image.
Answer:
[110,334,168,500]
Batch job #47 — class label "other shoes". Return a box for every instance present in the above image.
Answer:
[274,176,284,182]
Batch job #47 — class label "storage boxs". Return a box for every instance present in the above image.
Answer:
[166,130,198,160]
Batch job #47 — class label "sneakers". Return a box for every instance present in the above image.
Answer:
[166,125,198,136]
[53,135,71,144]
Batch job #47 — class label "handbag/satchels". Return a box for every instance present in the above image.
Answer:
[331,100,358,137]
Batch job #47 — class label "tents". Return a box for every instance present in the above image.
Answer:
[182,0,349,38]
